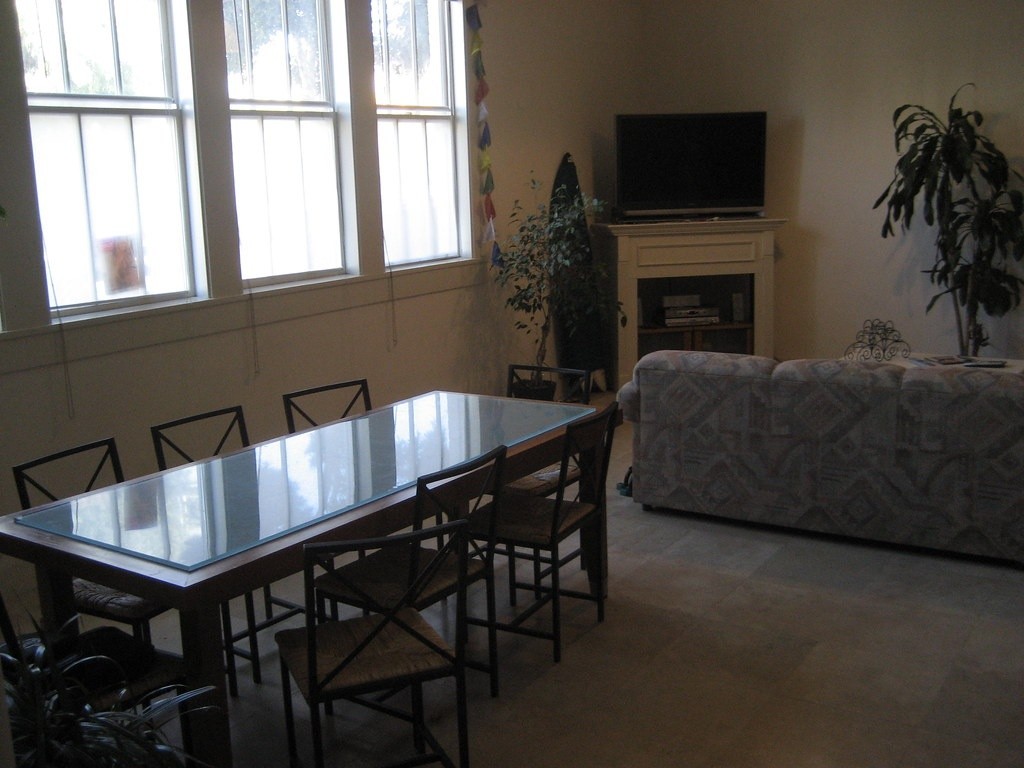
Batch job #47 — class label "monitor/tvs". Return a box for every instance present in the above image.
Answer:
[615,111,766,224]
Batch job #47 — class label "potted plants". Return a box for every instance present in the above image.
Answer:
[483,175,629,403]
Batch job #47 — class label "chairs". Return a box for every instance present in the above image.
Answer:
[12,365,593,768]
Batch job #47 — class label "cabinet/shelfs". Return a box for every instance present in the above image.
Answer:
[589,218,790,398]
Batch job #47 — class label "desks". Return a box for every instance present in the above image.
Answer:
[0,391,612,768]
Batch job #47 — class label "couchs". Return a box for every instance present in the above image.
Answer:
[614,351,1024,570]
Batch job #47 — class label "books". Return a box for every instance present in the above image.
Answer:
[731,278,744,321]
[655,293,720,327]
